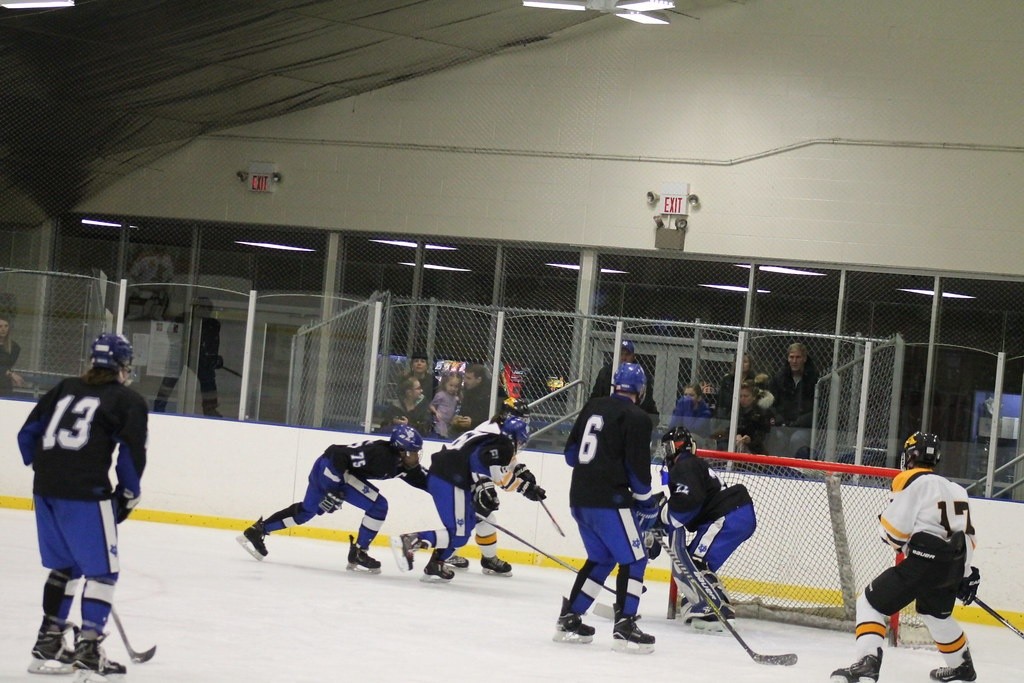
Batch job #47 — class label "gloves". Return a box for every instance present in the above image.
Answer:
[515,480,547,501]
[210,354,225,371]
[954,567,982,606]
[112,490,141,525]
[512,463,536,485]
[649,510,670,538]
[641,525,662,560]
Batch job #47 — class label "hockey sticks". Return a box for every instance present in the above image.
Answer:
[536,495,564,535]
[109,605,156,664]
[473,509,646,594]
[973,597,1024,638]
[650,527,797,666]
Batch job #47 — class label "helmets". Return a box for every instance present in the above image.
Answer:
[192,297,214,318]
[499,397,531,425]
[613,361,647,407]
[900,431,941,473]
[90,332,134,374]
[662,427,698,465]
[500,417,529,450]
[390,425,421,452]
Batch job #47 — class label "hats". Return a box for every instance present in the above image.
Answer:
[620,339,635,355]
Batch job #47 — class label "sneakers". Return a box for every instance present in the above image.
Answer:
[612,603,656,653]
[72,625,128,683]
[689,611,737,638]
[346,531,513,583]
[930,647,977,683]
[553,596,596,643]
[235,516,268,562]
[828,647,884,683]
[27,615,76,674]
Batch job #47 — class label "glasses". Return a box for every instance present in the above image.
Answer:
[411,385,423,391]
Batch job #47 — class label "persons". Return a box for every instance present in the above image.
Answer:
[429,370,462,441]
[831,431,981,683]
[589,340,661,430]
[0,317,25,398]
[123,251,175,319]
[451,364,504,434]
[658,426,756,626]
[16,333,149,675]
[154,297,224,418]
[769,343,825,428]
[396,351,440,432]
[556,361,658,645]
[244,425,430,569]
[714,350,766,419]
[446,397,547,573]
[708,379,771,457]
[378,375,433,437]
[401,417,530,578]
[668,383,712,448]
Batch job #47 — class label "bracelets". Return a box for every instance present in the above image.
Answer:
[7,371,13,378]
